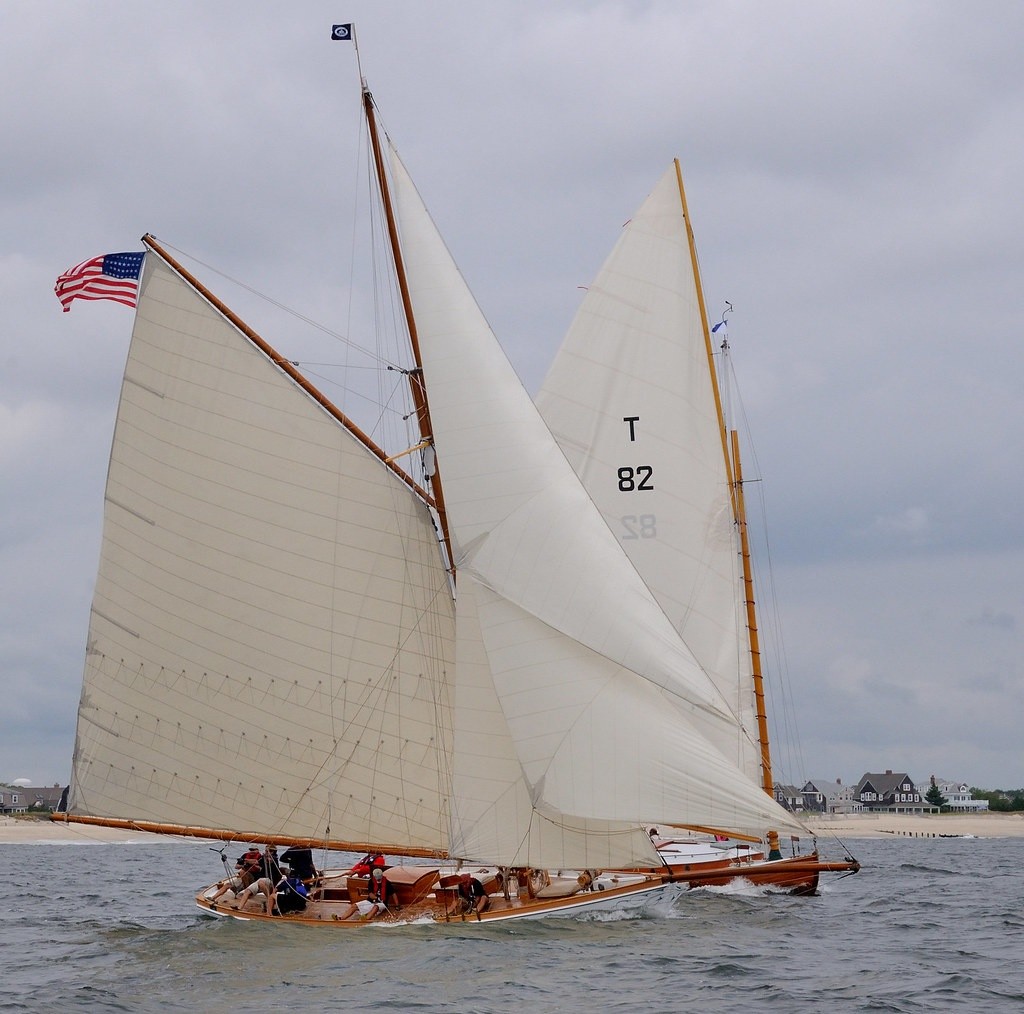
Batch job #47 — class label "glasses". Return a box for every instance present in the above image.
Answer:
[249,848,257,852]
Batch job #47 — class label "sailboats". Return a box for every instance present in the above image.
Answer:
[48,13,861,927]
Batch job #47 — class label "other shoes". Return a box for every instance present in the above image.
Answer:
[360,914,366,920]
[331,913,341,920]
[206,897,215,902]
[230,906,240,910]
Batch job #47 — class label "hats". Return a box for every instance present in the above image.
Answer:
[373,869,382,880]
[248,844,258,850]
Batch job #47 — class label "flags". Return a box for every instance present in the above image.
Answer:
[55,251,146,312]
[331,23,352,40]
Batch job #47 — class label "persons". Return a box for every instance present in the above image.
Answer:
[443,874,489,916]
[649,828,660,843]
[332,869,399,920]
[207,844,318,916]
[348,854,385,877]
